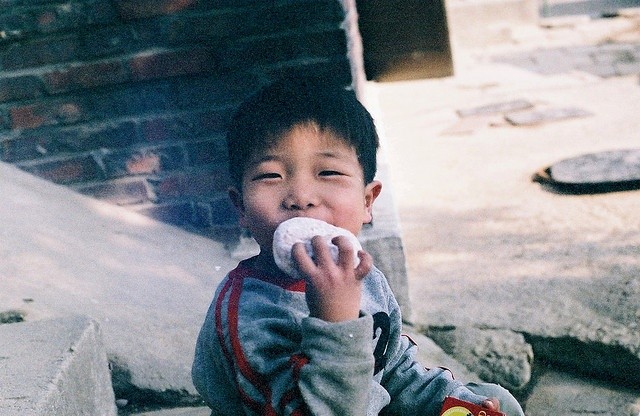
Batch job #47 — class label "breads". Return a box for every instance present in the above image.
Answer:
[272,216,364,279]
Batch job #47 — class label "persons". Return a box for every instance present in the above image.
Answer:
[191,81,523,415]
